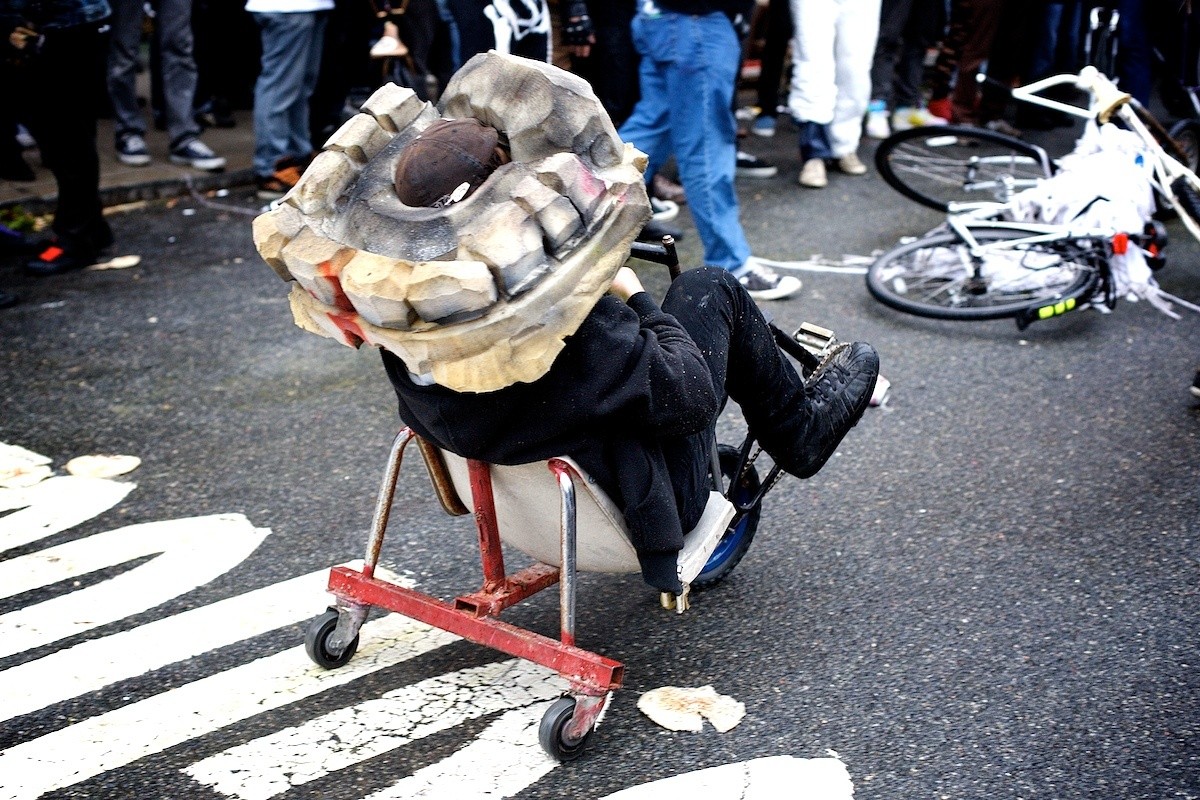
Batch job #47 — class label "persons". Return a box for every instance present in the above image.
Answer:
[379,128,880,598]
[730,0,796,178]
[790,0,881,187]
[621,0,804,300]
[245,0,334,198]
[0,0,248,273]
[867,0,1200,148]
[307,0,689,243]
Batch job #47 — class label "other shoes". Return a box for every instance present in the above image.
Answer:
[892,106,949,131]
[839,153,868,176]
[0,152,39,182]
[15,125,36,148]
[1188,369,1200,399]
[865,110,892,139]
[927,99,952,120]
[797,156,829,189]
[21,220,115,279]
[649,173,687,204]
[748,116,778,140]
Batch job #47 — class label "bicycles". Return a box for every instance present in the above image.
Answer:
[863,123,1168,338]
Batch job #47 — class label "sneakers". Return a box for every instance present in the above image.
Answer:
[648,196,681,226]
[729,255,803,302]
[113,132,154,168]
[167,133,228,173]
[734,150,779,180]
[781,341,881,480]
[256,156,301,193]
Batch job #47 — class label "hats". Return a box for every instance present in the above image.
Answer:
[392,115,509,209]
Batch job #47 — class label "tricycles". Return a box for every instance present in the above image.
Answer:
[305,231,890,763]
